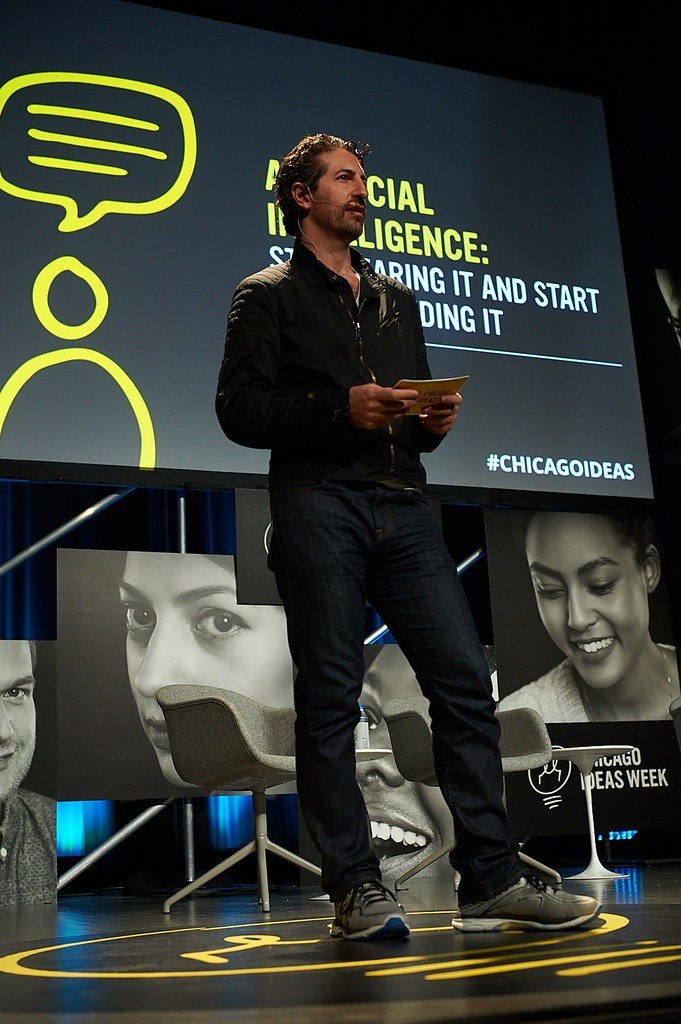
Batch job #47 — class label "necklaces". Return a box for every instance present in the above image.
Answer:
[583,647,673,722]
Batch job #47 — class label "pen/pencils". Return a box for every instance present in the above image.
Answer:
[378,312,400,332]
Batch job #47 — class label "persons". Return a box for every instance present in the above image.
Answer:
[494,510,681,722]
[355,644,456,881]
[0,639,58,906]
[119,551,297,795]
[215,134,605,939]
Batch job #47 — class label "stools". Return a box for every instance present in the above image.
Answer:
[309,748,392,901]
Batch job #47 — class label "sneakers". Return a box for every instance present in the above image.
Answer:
[450,872,601,934]
[328,877,412,941]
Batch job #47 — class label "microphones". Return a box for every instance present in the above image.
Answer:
[304,183,355,208]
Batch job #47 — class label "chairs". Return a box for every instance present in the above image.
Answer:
[381,695,563,891]
[157,683,300,914]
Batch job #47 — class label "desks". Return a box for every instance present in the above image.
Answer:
[549,745,634,881]
